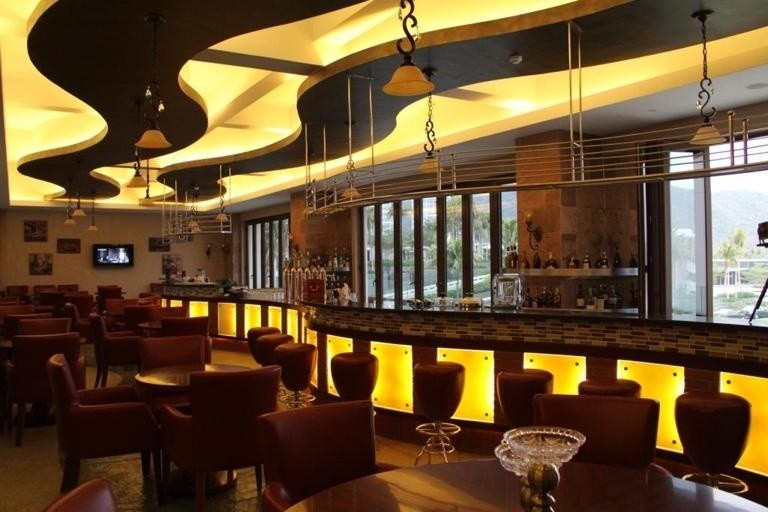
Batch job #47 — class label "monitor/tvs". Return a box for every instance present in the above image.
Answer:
[93,243,134,267]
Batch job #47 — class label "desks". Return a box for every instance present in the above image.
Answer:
[134,363,254,481]
[284,459,768,510]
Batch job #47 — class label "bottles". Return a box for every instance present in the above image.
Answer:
[289,246,350,268]
[325,275,349,305]
[629,281,637,307]
[504,245,637,269]
[577,281,624,311]
[524,286,561,309]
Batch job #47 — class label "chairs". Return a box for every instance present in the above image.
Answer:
[2,283,212,449]
[531,394,660,462]
[49,478,117,511]
[157,365,281,510]
[48,352,157,493]
[255,400,376,511]
[139,334,204,370]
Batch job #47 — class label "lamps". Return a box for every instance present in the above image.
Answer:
[337,117,366,202]
[133,12,177,153]
[682,8,731,146]
[412,63,447,177]
[59,95,231,236]
[377,0,435,99]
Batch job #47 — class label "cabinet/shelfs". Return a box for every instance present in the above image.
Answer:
[503,267,641,316]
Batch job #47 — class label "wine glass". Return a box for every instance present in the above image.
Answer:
[494,424,587,511]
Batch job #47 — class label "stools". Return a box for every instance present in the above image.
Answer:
[330,352,379,416]
[248,327,282,368]
[411,362,465,465]
[496,368,553,429]
[577,378,643,396]
[276,343,317,407]
[675,390,752,495]
[256,334,295,367]
